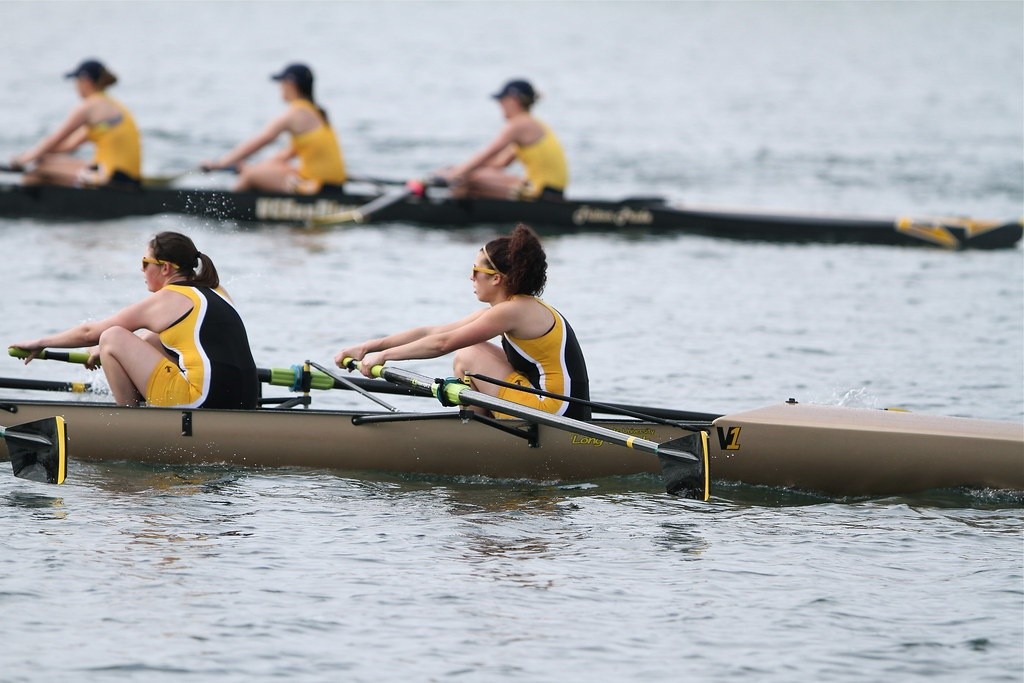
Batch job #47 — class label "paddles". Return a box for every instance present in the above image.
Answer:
[0,377,92,394]
[590,398,729,422]
[7,344,435,399]
[0,416,69,486]
[307,165,449,234]
[201,160,446,187]
[340,354,713,503]
[0,162,144,206]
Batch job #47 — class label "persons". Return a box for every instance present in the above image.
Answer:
[8,232,261,410]
[7,62,139,189]
[436,79,567,202]
[199,63,346,199]
[333,226,592,421]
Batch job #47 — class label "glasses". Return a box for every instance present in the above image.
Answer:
[139,256,180,272]
[471,264,498,278]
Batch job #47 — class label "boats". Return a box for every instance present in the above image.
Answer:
[0,164,1024,252]
[1,347,1023,501]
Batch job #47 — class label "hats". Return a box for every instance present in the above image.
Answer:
[491,81,536,100]
[269,64,314,85]
[65,61,106,82]
